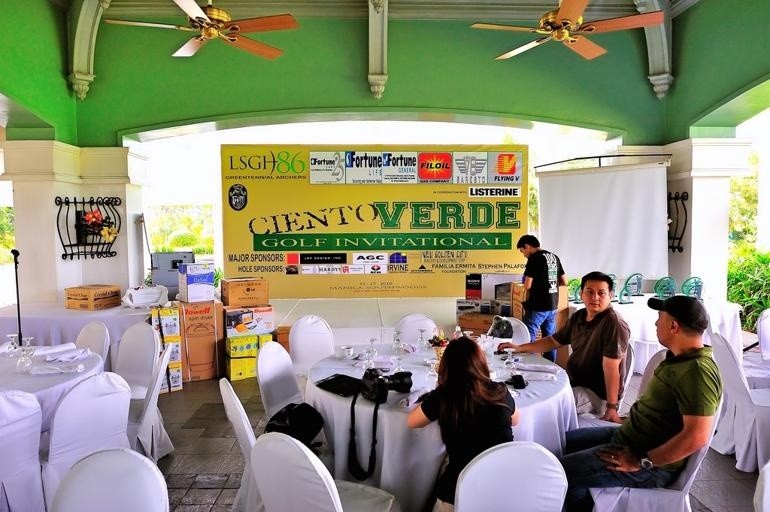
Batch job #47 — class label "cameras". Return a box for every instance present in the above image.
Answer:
[361,367,412,404]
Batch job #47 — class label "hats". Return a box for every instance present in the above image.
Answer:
[647,294,710,333]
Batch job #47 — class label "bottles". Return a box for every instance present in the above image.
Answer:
[452,326,496,363]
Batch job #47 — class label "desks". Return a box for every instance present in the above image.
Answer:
[0,343,104,432]
[301,337,575,509]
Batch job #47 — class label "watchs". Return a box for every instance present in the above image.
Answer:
[606,402,619,411]
[639,450,653,470]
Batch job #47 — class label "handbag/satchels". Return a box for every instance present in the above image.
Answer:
[262,401,324,449]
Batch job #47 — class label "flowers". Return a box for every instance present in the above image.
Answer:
[72,209,121,243]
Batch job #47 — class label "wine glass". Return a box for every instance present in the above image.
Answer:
[505,347,519,375]
[363,325,440,389]
[3,334,37,376]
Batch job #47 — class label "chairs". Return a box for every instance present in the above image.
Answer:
[755,307,770,361]
[588,387,723,511]
[630,333,665,376]
[74,320,111,365]
[711,301,770,387]
[394,313,437,345]
[583,344,636,418]
[254,341,299,413]
[752,461,770,512]
[114,322,162,400]
[289,313,335,378]
[49,447,171,512]
[710,332,770,471]
[446,440,569,511]
[217,377,260,511]
[486,316,531,347]
[248,430,394,512]
[1,390,46,511]
[38,371,132,508]
[578,349,668,426]
[126,342,176,461]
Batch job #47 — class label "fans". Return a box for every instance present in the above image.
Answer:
[103,0,301,64]
[468,0,666,63]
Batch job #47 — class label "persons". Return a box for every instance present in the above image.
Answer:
[407,336,521,512]
[517,234,568,364]
[556,296,723,512]
[497,271,632,425]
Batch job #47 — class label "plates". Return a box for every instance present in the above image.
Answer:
[334,352,360,360]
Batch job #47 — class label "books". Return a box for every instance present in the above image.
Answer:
[131,286,168,307]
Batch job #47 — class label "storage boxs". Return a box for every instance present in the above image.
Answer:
[176,261,215,304]
[558,284,570,312]
[220,275,271,308]
[493,281,525,303]
[150,250,196,290]
[64,283,123,310]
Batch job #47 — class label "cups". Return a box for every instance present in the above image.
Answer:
[334,343,353,358]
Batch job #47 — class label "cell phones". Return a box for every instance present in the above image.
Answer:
[512,375,525,389]
